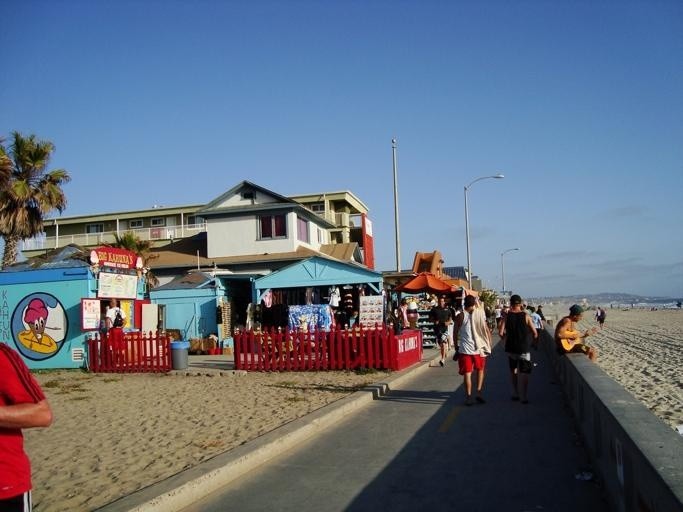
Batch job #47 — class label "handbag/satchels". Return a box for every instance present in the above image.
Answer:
[519,358,531,373]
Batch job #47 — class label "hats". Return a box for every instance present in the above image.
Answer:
[569,304,584,313]
[510,295,521,304]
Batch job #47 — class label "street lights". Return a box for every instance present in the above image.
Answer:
[464,173,520,296]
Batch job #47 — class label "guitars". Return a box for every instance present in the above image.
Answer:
[561,324,607,351]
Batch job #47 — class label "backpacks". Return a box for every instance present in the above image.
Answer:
[110,309,123,327]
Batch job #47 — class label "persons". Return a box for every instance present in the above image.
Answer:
[520,303,547,350]
[407,309,421,328]
[499,294,539,405]
[554,303,596,361]
[453,295,492,405]
[105,299,127,367]
[429,295,510,367]
[397,303,409,328]
[1,341,52,512]
[595,307,606,330]
[343,307,358,330]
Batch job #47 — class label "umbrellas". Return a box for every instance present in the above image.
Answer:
[391,271,460,295]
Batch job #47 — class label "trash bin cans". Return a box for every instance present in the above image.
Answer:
[547,320,553,328]
[171,341,190,369]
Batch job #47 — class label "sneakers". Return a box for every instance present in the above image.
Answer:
[439,360,445,367]
[465,398,473,405]
[475,396,486,404]
